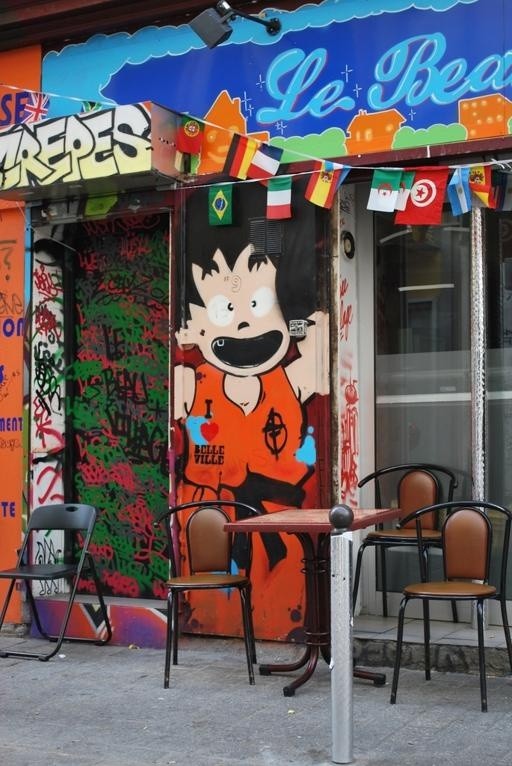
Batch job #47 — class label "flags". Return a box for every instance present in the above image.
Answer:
[172,118,497,225]
[22,91,50,123]
[78,100,103,113]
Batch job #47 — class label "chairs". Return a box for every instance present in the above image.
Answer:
[0,504,112,661]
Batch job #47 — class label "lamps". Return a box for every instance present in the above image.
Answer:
[189,1,280,49]
